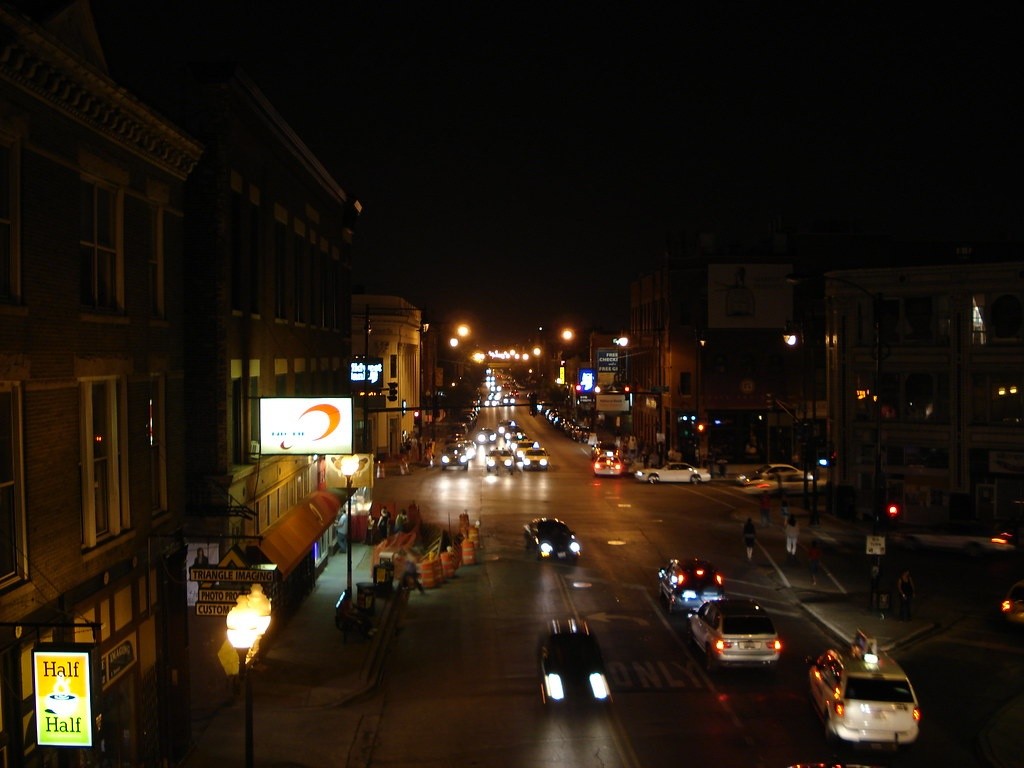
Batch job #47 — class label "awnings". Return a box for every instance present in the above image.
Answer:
[245,487,359,582]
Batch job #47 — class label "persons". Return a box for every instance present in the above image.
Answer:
[333,509,347,554]
[781,495,789,526]
[742,517,756,562]
[760,491,772,527]
[786,513,799,561]
[406,436,435,467]
[898,569,912,624]
[808,540,820,585]
[194,548,209,588]
[622,432,727,479]
[364,506,408,546]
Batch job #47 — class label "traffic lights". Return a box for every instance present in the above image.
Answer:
[887,504,899,516]
[387,382,398,402]
[698,424,704,430]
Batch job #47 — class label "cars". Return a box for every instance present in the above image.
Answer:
[657,562,725,613]
[440,369,591,475]
[524,518,580,560]
[805,647,920,744]
[634,462,711,485]
[538,624,608,706]
[685,598,781,672]
[591,441,620,460]
[1003,580,1024,623]
[593,453,622,477]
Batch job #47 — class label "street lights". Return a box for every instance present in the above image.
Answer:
[431,324,469,440]
[338,453,360,607]
[781,316,822,527]
[563,329,594,431]
[223,601,272,768]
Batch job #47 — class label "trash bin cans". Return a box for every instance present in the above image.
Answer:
[375,561,395,592]
[356,582,375,618]
